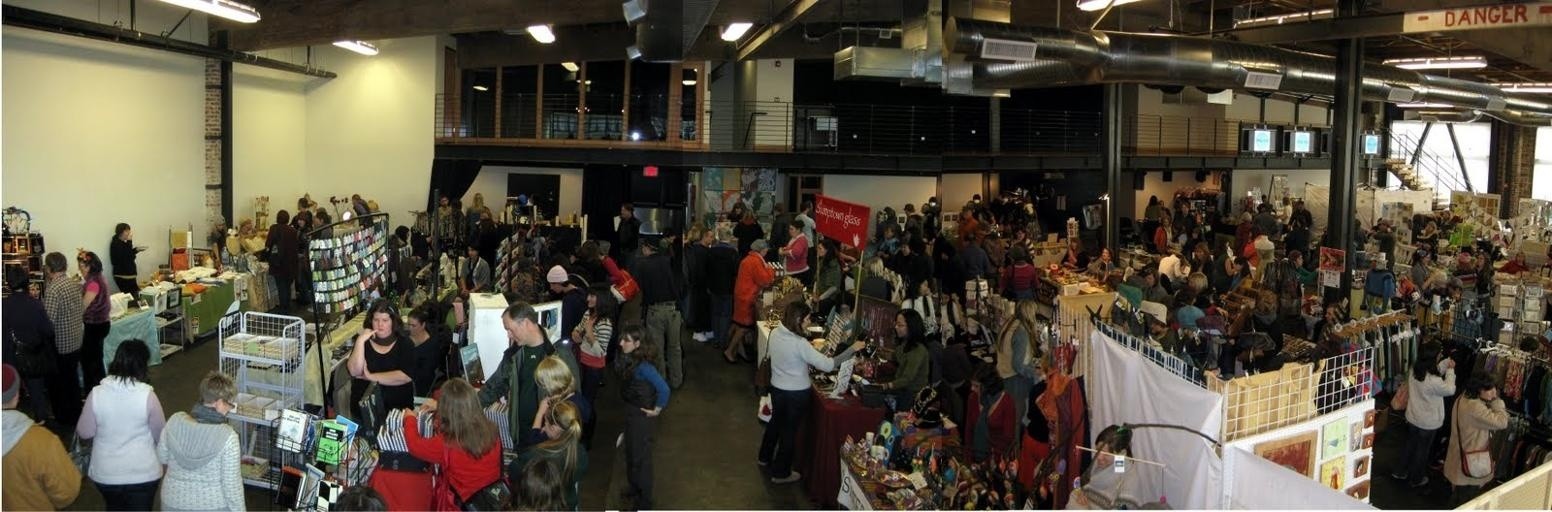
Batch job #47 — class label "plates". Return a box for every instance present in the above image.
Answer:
[885,488,922,510]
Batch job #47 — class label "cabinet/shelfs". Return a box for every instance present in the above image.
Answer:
[138,287,184,361]
[217,310,307,491]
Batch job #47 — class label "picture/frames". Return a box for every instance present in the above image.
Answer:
[1254,430,1318,486]
[155,287,182,314]
[2,234,45,301]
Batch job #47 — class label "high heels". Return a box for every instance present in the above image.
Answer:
[735,350,753,363]
[721,352,738,365]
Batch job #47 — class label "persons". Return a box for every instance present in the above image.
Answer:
[721,239,776,364]
[792,200,816,290]
[777,218,810,289]
[727,200,751,223]
[156,193,717,512]
[768,201,792,248]
[42,252,86,416]
[26,277,43,305]
[110,222,150,308]
[72,338,167,512]
[76,248,114,402]
[733,210,765,254]
[2,363,83,510]
[812,204,1552,512]
[1,262,56,425]
[754,301,866,486]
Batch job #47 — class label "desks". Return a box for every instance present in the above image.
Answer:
[103,306,157,378]
[181,262,270,343]
[810,381,886,498]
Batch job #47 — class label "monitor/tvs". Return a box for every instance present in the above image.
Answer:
[1289,130,1315,155]
[1360,134,1382,156]
[1247,130,1278,154]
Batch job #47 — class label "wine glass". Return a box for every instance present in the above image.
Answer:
[872,470,913,494]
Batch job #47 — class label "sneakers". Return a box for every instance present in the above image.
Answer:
[621,485,637,496]
[634,501,652,510]
[757,458,771,468]
[772,471,799,483]
[1392,471,1430,489]
[693,329,730,349]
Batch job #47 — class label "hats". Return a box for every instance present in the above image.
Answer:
[547,265,569,283]
[2,364,22,402]
[642,236,661,251]
[1168,243,1183,256]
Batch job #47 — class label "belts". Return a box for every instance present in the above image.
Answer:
[652,302,674,306]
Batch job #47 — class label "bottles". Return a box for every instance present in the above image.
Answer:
[237,254,246,272]
[220,246,231,263]
[862,328,876,357]
[439,251,448,274]
[535,210,544,225]
[767,260,784,279]
[864,431,888,466]
[565,208,578,224]
[309,221,389,314]
[840,305,851,319]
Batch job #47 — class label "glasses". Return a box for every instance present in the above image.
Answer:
[894,321,908,327]
[226,401,238,412]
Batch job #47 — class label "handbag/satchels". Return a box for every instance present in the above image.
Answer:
[755,357,771,396]
[1392,379,1409,412]
[260,250,271,259]
[1420,288,1434,307]
[1237,330,1275,352]
[571,341,581,359]
[461,480,514,511]
[66,431,94,481]
[1461,450,1495,479]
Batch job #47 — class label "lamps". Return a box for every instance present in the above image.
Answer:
[335,40,379,57]
[472,71,491,92]
[1382,56,1552,95]
[152,0,262,24]
